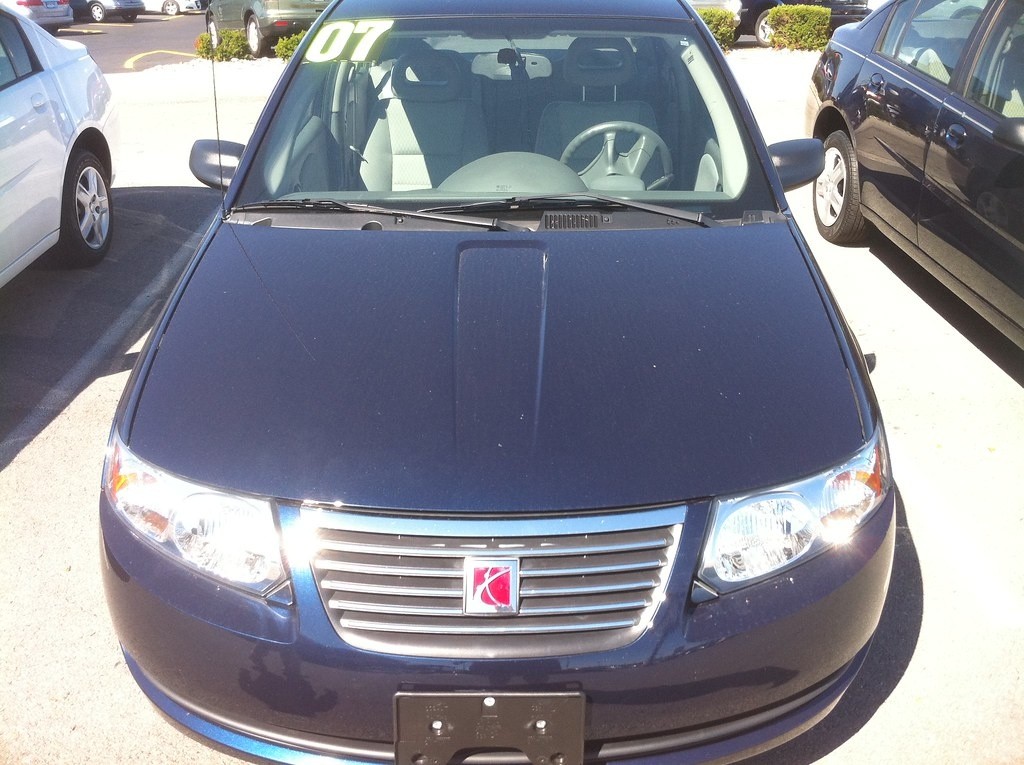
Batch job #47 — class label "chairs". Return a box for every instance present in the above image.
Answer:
[354,50,489,193]
[893,45,949,90]
[993,35,1024,118]
[538,35,670,191]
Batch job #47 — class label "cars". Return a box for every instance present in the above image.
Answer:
[686,0,877,49]
[807,0,1024,351]
[69,0,146,22]
[94,0,895,764]
[866,1,990,21]
[0,11,116,291]
[142,0,201,15]
[0,1,73,34]
[204,1,332,57]
